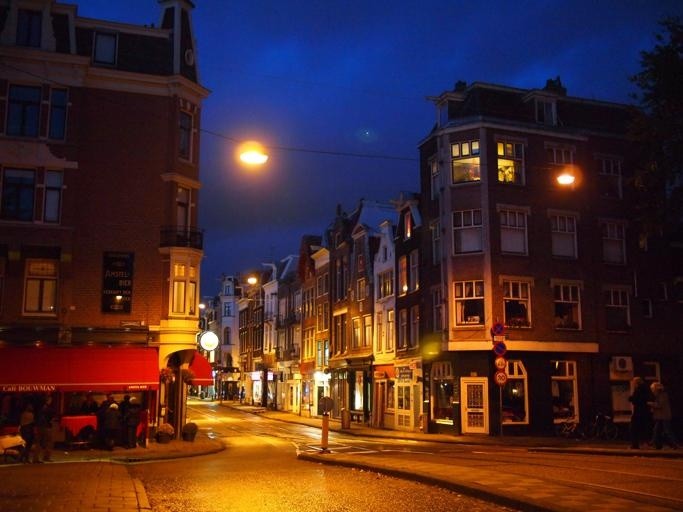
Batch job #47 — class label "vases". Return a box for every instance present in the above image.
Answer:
[157,431,171,444]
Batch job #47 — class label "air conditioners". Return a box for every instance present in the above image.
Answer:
[611,356,634,373]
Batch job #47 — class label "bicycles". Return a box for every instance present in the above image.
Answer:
[565,409,620,443]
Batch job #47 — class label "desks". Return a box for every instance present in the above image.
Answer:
[59,414,98,452]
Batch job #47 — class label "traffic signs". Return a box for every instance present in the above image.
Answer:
[494,371,508,386]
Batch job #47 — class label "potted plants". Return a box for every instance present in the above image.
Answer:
[181,422,197,442]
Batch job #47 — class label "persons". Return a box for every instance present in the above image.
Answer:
[19,394,132,464]
[648,382,678,451]
[628,376,655,449]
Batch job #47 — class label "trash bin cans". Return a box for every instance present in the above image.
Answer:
[341,408,351,429]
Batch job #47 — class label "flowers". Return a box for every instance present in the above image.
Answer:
[159,368,176,384]
[155,422,174,437]
